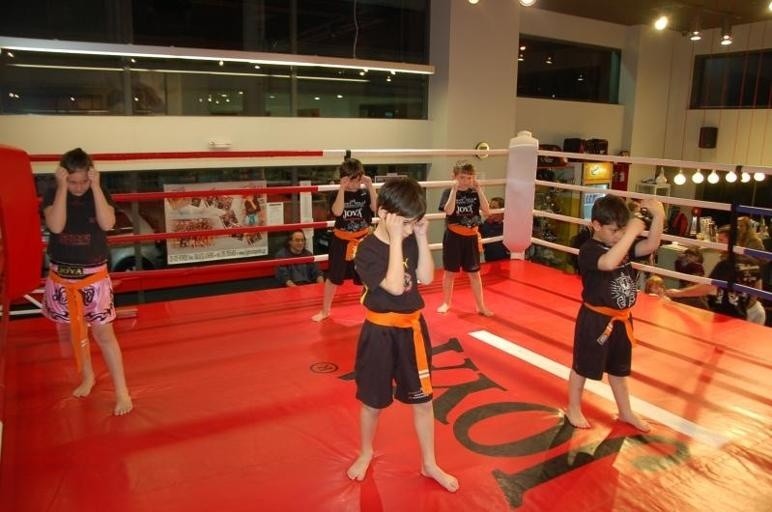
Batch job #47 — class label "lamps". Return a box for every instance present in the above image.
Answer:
[688,8,734,47]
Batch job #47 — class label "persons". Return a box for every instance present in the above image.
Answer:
[481,194,508,261]
[346,174,459,492]
[40,146,135,418]
[308,149,378,323]
[644,275,667,300]
[437,159,495,318]
[560,192,667,432]
[668,202,772,325]
[167,184,264,250]
[273,229,327,286]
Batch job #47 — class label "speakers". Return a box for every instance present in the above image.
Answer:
[699,127,717,147]
